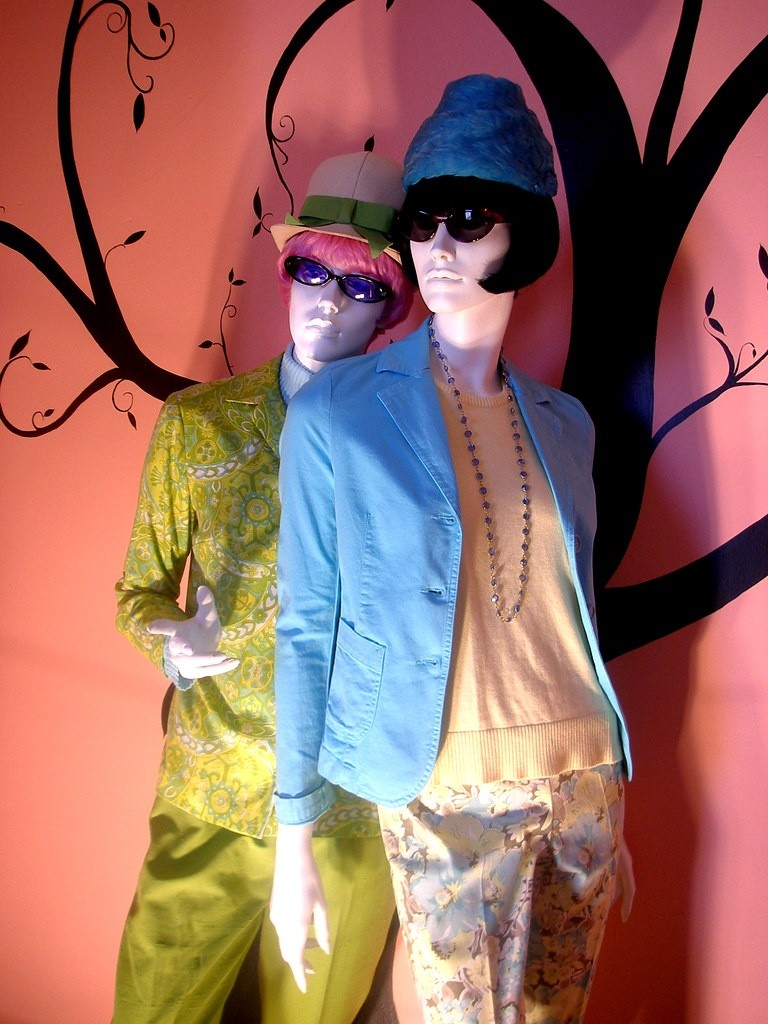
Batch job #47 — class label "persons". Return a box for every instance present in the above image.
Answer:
[268,76,637,1024]
[114,135,413,1024]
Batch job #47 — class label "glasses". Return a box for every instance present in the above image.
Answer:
[284,256,394,303]
[394,206,510,243]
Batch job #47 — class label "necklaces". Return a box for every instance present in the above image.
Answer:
[421,312,531,621]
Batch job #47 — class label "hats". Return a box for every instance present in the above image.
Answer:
[270,151,407,267]
[401,73,558,196]
